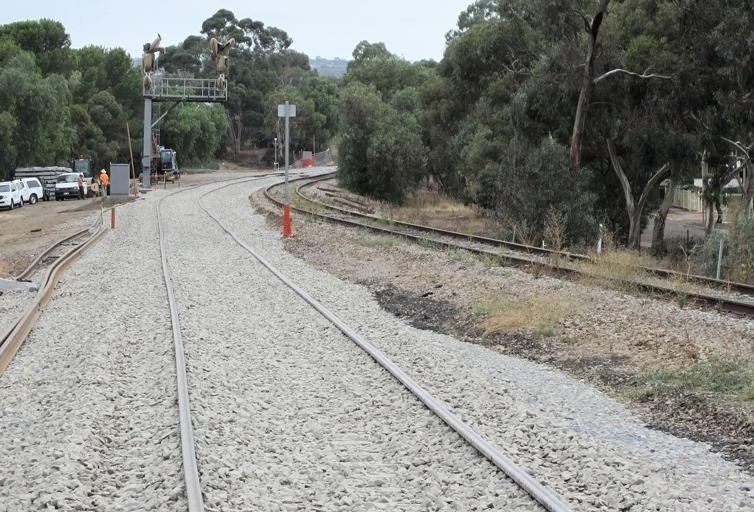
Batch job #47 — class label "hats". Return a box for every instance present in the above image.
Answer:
[101,169,106,173]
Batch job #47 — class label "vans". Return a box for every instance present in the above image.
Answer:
[55,172,87,200]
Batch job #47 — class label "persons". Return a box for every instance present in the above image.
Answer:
[77,172,85,200]
[99,169,109,199]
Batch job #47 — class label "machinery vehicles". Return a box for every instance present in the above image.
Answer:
[72,157,94,179]
[139,146,180,182]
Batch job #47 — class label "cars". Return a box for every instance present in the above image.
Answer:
[0,177,43,210]
